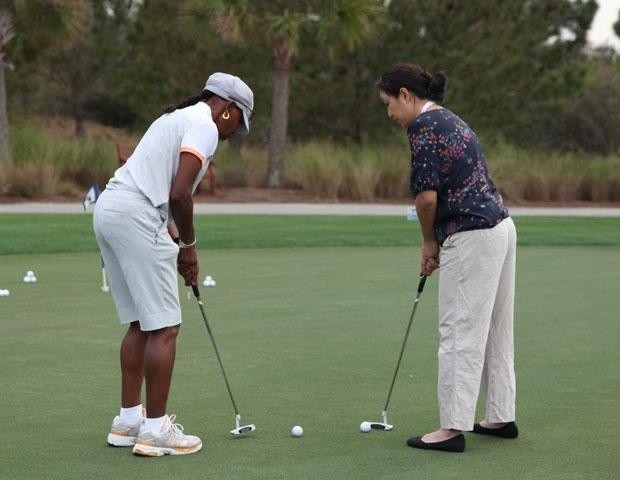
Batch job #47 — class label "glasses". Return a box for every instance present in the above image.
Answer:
[228,96,256,122]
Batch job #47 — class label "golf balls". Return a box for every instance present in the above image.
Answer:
[202,275,216,286]
[0,288,10,298]
[22,271,37,283]
[361,421,371,433]
[292,425,302,438]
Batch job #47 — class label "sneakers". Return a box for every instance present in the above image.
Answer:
[133,416,203,457]
[406,434,466,453]
[470,421,518,438]
[106,408,145,447]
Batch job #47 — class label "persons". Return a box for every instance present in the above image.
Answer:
[374,62,520,455]
[91,71,258,458]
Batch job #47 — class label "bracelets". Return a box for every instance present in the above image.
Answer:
[172,236,181,243]
[177,236,198,248]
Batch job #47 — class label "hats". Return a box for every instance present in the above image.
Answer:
[203,72,255,135]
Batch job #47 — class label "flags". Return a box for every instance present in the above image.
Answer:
[82,183,100,210]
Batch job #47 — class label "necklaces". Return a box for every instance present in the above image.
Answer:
[421,101,436,113]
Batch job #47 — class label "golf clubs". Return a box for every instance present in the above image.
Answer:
[190,283,255,434]
[369,274,427,432]
